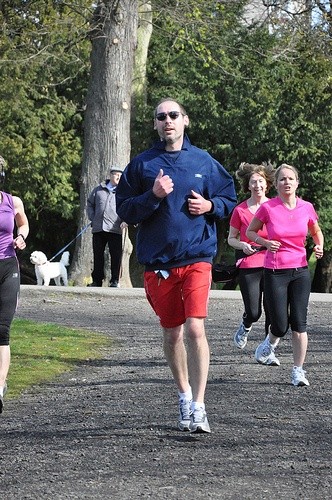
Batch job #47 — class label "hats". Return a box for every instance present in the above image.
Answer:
[109,167,124,173]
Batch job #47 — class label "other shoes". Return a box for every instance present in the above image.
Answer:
[87,282,102,287]
[0,395,5,414]
[3,382,7,398]
[109,281,118,287]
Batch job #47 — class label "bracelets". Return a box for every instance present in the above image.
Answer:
[255,237,262,243]
[18,234,24,240]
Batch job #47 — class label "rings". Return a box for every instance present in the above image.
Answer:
[321,256,323,258]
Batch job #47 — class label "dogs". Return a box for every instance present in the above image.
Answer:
[30,250,71,287]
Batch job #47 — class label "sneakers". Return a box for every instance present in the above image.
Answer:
[234,321,253,349]
[255,333,280,363]
[266,353,280,366]
[188,407,211,433]
[291,366,309,386]
[178,395,193,432]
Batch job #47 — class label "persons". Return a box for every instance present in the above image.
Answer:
[0,156,30,414]
[115,98,239,434]
[87,167,129,288]
[228,161,273,350]
[246,163,324,387]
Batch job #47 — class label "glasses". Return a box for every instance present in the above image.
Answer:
[155,112,185,122]
[112,172,122,178]
